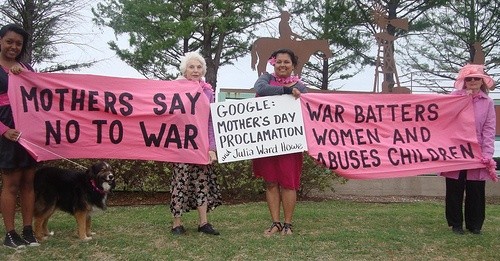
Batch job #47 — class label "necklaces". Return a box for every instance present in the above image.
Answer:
[271,71,299,83]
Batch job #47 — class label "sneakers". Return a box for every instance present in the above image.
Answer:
[172,225,184,235]
[198,223,219,235]
[23,225,39,246]
[3,231,26,248]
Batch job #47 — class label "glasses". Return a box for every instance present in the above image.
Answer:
[466,77,481,81]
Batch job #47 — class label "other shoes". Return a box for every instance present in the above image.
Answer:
[468,229,480,234]
[452,226,463,234]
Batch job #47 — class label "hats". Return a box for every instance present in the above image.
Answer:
[454,65,494,90]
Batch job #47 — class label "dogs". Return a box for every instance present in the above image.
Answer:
[29,160,114,242]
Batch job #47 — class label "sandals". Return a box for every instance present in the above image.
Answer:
[269,221,283,233]
[281,223,293,235]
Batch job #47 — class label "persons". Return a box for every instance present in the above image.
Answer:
[441,65,496,234]
[170,52,221,235]
[0,23,42,249]
[253,49,311,237]
